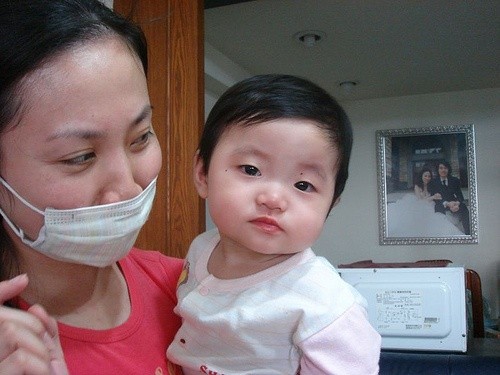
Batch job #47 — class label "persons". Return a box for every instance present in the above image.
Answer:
[426,159,470,235]
[159,72,382,375]
[387,168,445,236]
[1,0,184,374]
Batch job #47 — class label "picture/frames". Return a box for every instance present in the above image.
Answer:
[375,123,479,246]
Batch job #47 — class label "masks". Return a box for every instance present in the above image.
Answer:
[0,177,157,268]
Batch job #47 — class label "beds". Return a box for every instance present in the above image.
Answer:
[337,258,488,350]
